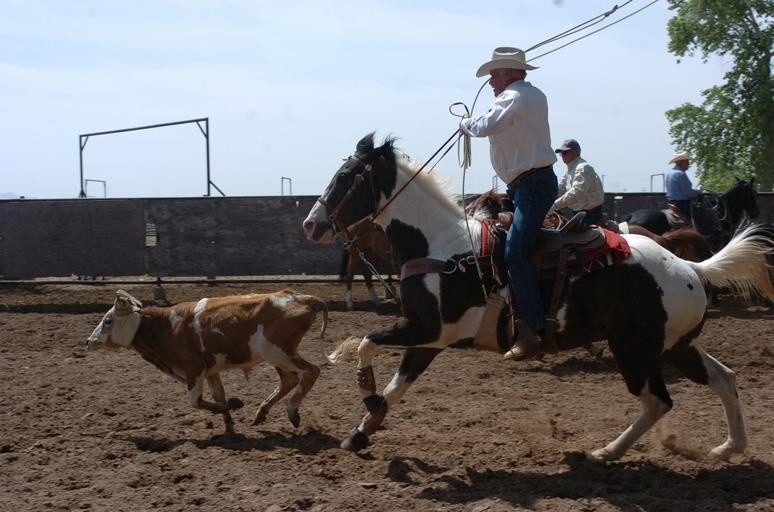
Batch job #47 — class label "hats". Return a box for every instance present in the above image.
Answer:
[555,138,580,153]
[476,46,540,77]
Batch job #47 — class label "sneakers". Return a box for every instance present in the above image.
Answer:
[503,334,545,361]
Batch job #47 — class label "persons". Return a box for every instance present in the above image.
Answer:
[337,248,348,281]
[460,45,560,361]
[664,153,712,233]
[545,136,604,225]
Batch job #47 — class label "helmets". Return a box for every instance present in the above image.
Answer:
[669,151,695,162]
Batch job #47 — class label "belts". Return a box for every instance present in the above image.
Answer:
[507,164,553,190]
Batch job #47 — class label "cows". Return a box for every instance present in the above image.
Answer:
[84,288,329,438]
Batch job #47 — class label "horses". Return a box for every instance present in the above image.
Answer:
[301,132,774,461]
